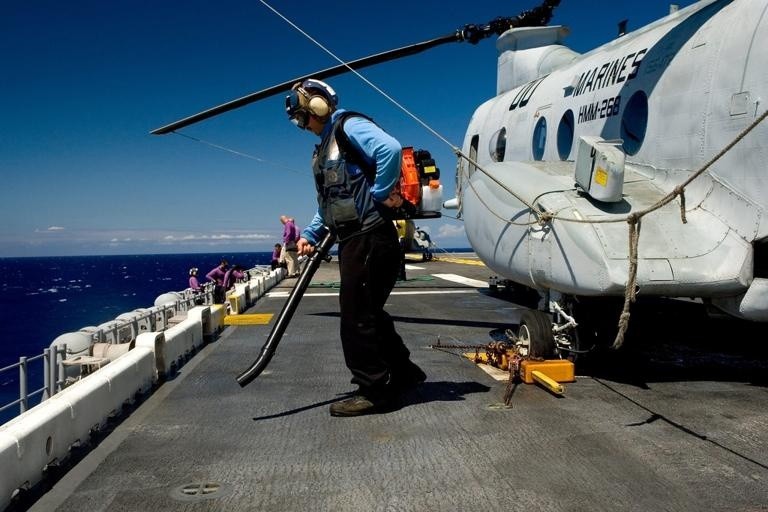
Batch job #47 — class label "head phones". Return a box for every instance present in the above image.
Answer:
[292,82,332,124]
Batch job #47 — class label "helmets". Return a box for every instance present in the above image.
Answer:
[283,77,339,117]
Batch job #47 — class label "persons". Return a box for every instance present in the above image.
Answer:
[284,78,428,418]
[288,217,300,244]
[270,242,282,272]
[204,259,229,304]
[222,263,245,300]
[279,215,301,279]
[188,267,204,305]
[390,219,415,284]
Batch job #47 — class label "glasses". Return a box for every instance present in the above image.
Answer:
[288,108,310,131]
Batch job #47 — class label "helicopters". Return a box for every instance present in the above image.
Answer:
[150,1,767,358]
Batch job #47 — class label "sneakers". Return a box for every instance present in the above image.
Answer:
[397,360,428,387]
[329,395,377,417]
[285,272,300,279]
